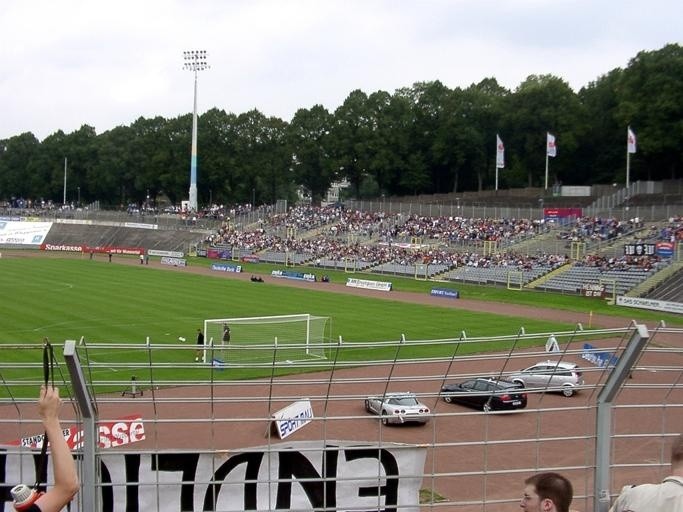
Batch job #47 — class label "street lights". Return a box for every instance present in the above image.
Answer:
[182,49,210,215]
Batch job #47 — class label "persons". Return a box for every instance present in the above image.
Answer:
[0,191,683,298]
[221,323,232,351]
[194,329,205,361]
[604,431,683,512]
[20,384,81,511]
[519,472,573,512]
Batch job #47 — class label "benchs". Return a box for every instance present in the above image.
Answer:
[199,213,683,304]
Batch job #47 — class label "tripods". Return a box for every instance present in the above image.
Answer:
[122,376,143,398]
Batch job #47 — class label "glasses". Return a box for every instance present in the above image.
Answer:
[523,495,542,501]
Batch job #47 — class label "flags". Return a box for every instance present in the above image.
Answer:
[497,134,505,169]
[546,132,558,158]
[628,127,637,154]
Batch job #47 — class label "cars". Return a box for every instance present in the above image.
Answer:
[508,359,584,397]
[440,377,527,411]
[365,391,431,427]
